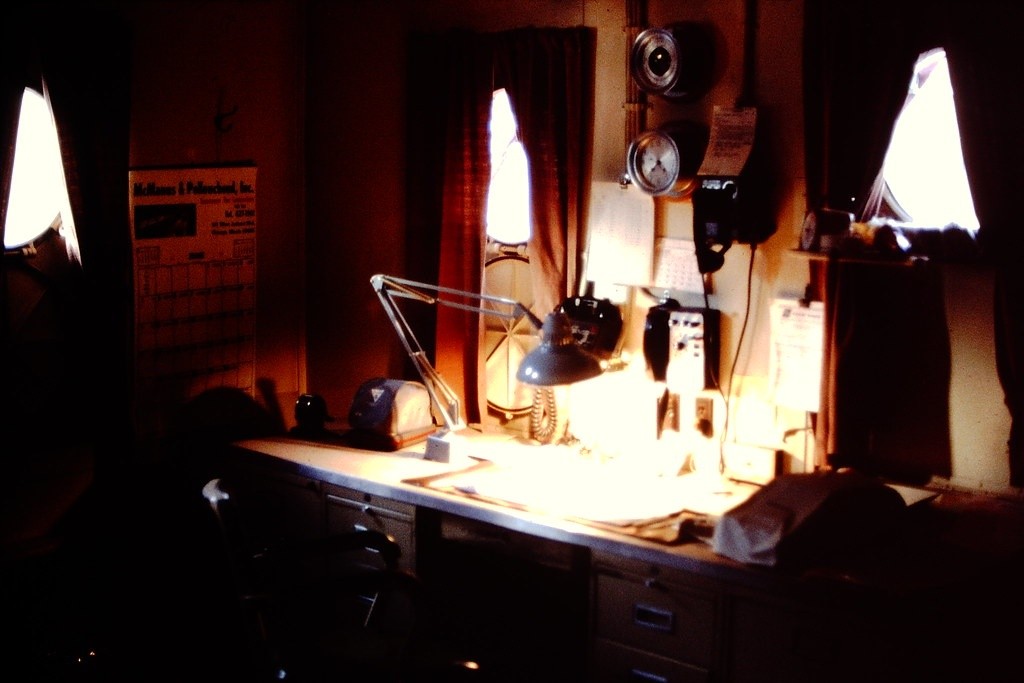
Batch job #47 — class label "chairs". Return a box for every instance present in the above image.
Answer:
[200,470,426,682]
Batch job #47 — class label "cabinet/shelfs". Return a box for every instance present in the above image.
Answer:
[230,405,940,683]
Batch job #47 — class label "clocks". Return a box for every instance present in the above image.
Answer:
[627,120,705,203]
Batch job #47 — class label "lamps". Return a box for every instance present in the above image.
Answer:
[369,274,604,462]
